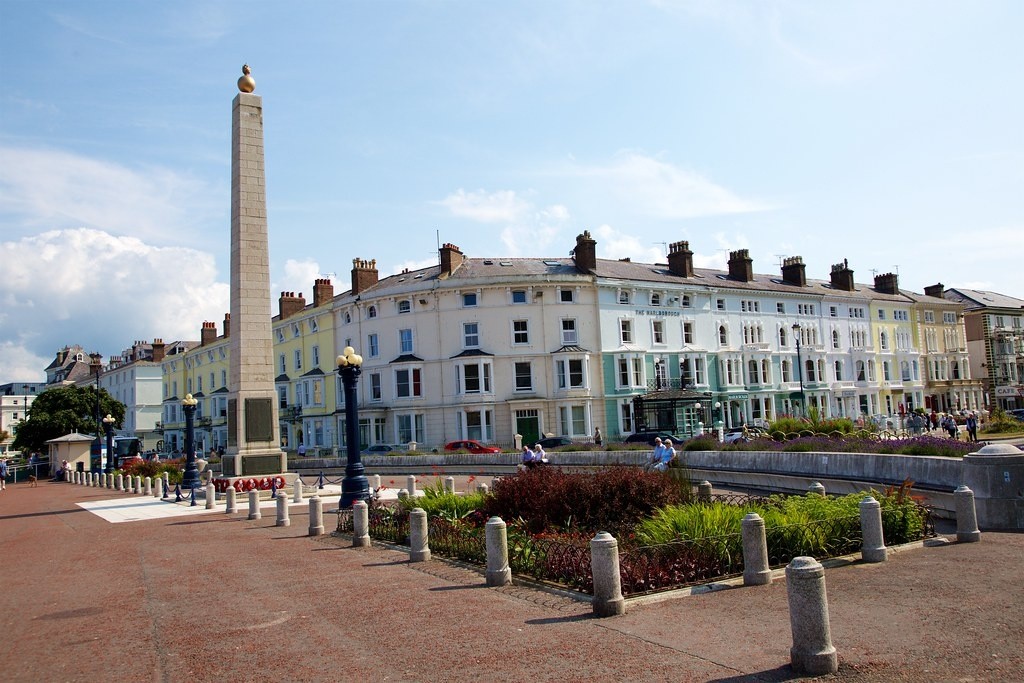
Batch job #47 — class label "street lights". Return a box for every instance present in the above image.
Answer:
[181,393,202,489]
[336,347,370,510]
[103,414,115,474]
[695,403,704,436]
[24,396,27,422]
[791,321,805,419]
[715,402,724,443]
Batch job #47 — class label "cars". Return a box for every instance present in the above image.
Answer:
[1005,409,1024,422]
[624,432,683,446]
[143,453,168,462]
[195,450,210,459]
[528,437,594,450]
[725,426,768,443]
[443,440,501,454]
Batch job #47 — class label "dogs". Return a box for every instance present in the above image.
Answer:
[28,475,37,487]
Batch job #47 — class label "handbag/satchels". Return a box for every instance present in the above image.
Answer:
[957,429,962,435]
[967,426,971,431]
[541,458,549,463]
[669,459,680,468]
[945,425,949,430]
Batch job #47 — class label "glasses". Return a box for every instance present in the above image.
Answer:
[655,441,661,443]
[665,443,670,444]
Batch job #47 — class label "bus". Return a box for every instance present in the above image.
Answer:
[104,436,144,465]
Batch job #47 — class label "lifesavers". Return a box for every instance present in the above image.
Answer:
[222,479,230,493]
[247,479,259,490]
[215,479,222,491]
[212,478,214,483]
[259,477,272,489]
[234,479,247,492]
[273,476,285,488]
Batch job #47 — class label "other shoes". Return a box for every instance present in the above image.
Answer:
[0,486,6,491]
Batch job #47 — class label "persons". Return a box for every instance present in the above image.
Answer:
[0,448,71,490]
[593,426,602,445]
[297,442,306,457]
[520,444,545,471]
[742,419,770,440]
[644,437,676,473]
[854,409,981,443]
[136,447,217,465]
